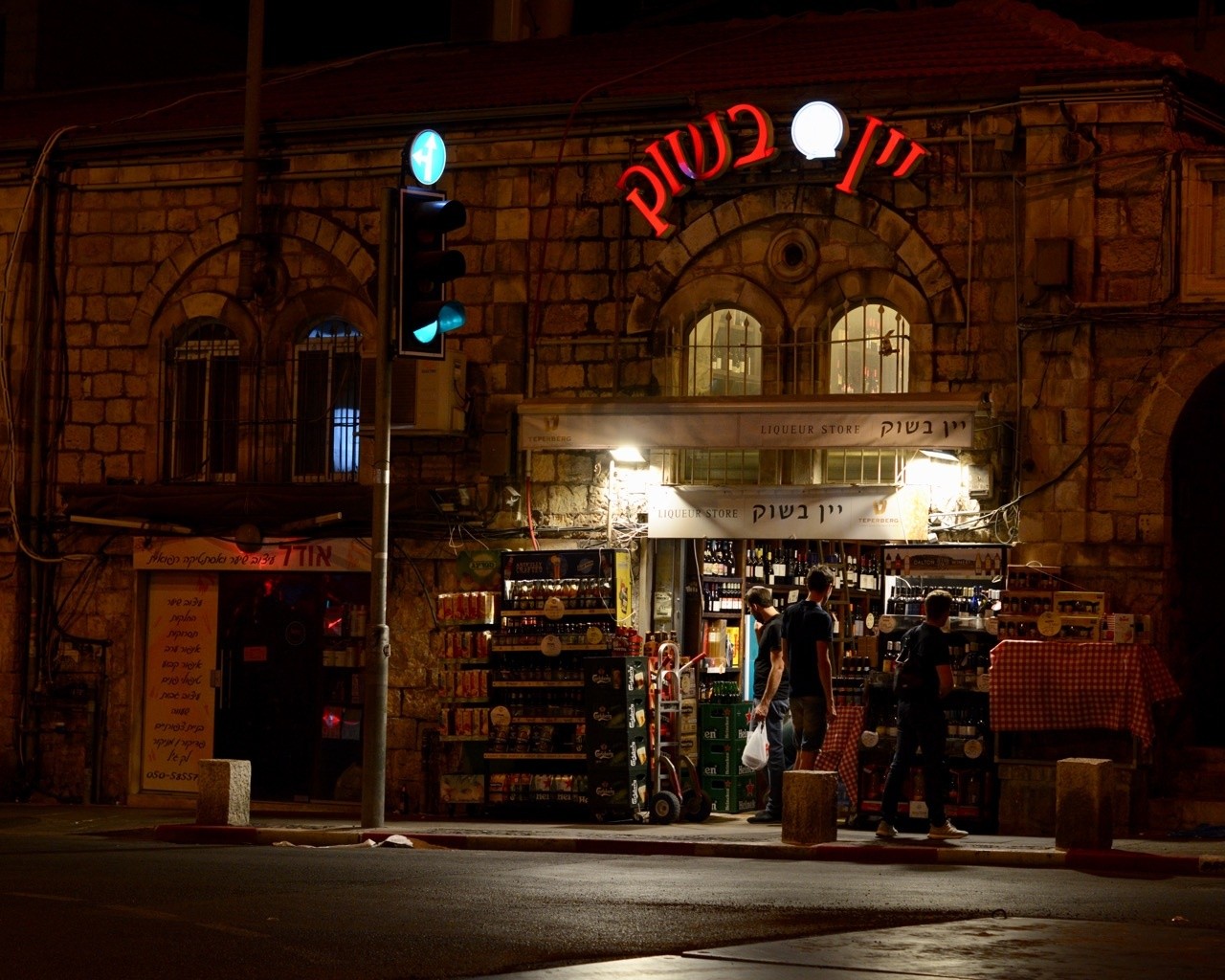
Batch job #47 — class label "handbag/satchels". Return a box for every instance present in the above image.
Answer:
[741,716,769,772]
[892,663,909,696]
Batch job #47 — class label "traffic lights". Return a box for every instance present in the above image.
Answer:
[397,189,467,361]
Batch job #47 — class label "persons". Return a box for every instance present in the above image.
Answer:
[782,717,797,772]
[743,585,790,823]
[782,563,839,772]
[876,591,969,840]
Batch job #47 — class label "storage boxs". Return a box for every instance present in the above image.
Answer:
[587,653,650,819]
[700,741,756,774]
[700,702,758,741]
[996,563,1153,645]
[703,775,757,811]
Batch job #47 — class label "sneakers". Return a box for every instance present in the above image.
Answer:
[875,821,898,836]
[927,818,969,840]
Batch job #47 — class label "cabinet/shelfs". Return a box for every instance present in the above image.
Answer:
[688,541,1011,816]
[482,548,636,816]
[433,624,496,809]
[316,634,363,799]
[712,323,761,395]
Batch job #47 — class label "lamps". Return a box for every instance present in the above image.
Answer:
[918,448,959,462]
[609,445,648,463]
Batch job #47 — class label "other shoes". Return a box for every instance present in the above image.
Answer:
[746,811,778,824]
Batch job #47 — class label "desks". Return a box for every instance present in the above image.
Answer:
[989,639,1177,774]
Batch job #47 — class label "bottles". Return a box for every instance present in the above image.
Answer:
[644,630,681,657]
[864,703,992,806]
[886,551,891,575]
[894,550,901,575]
[710,680,742,703]
[509,577,612,610]
[702,540,742,614]
[499,616,640,656]
[345,638,366,667]
[493,654,584,682]
[999,574,1100,639]
[883,640,991,688]
[499,690,585,717]
[575,724,586,753]
[904,552,910,575]
[975,549,1001,575]
[744,545,880,706]
[322,604,369,637]
[886,585,997,629]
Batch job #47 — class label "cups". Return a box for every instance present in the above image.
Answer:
[323,650,345,667]
